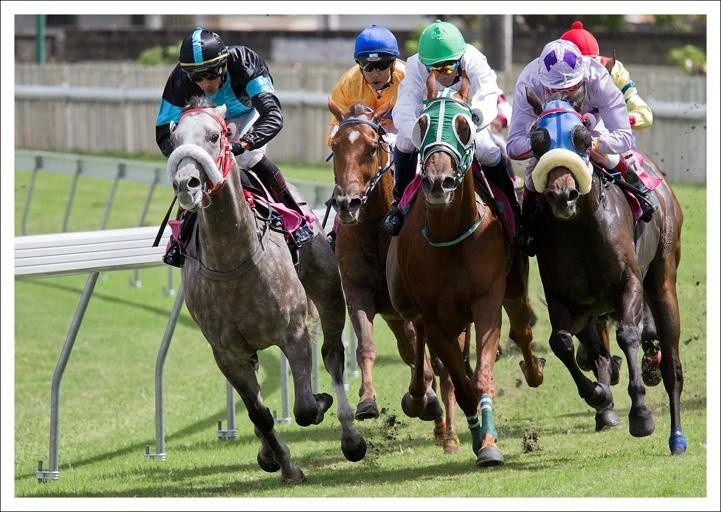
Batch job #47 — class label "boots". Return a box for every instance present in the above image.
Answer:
[483,154,522,237]
[520,182,543,258]
[382,145,417,236]
[249,155,313,245]
[612,154,658,219]
[164,206,196,268]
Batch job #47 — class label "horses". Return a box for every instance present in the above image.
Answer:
[326,49,686,469]
[168,93,368,487]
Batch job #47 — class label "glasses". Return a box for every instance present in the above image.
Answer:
[431,62,460,74]
[186,68,222,80]
[360,61,391,71]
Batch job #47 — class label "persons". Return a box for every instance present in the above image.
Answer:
[492,89,516,179]
[382,19,527,245]
[326,26,406,251]
[560,20,652,129]
[156,29,313,267]
[506,39,658,257]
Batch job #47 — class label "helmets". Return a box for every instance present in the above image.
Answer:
[179,29,229,73]
[537,21,599,89]
[418,20,466,65]
[353,24,399,60]
